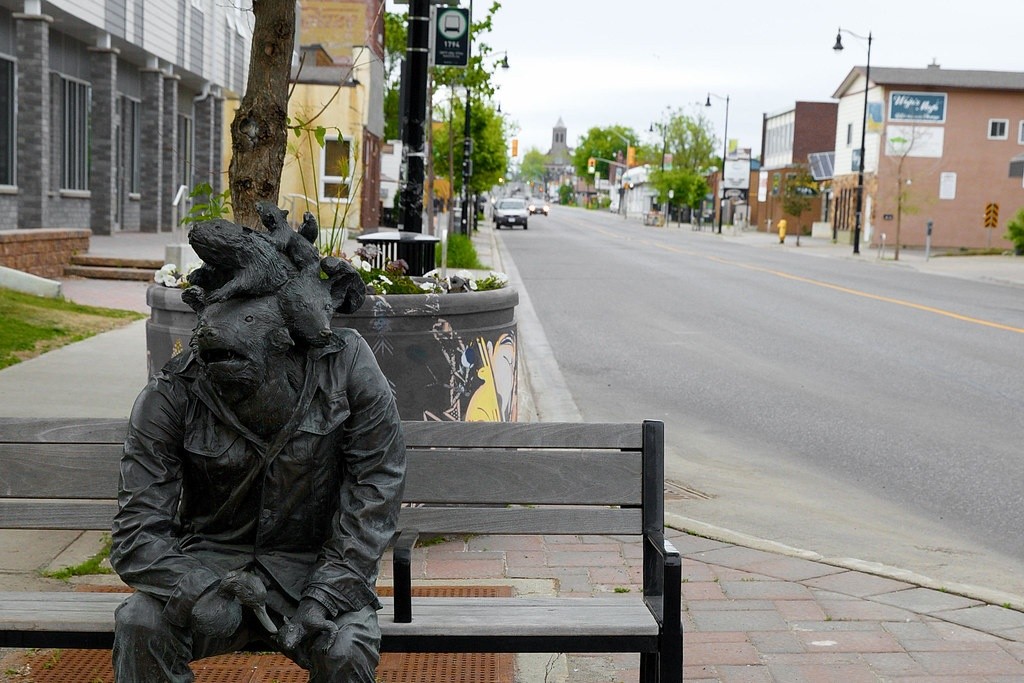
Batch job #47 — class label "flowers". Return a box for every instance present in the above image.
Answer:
[327,238,447,295]
[152,256,205,288]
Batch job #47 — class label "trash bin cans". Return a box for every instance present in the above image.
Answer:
[356,232,441,277]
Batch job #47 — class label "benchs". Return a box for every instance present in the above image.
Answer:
[0,419,684,683]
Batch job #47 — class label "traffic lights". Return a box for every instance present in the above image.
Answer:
[588,157,596,173]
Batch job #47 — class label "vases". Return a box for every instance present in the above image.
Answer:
[143,283,518,549]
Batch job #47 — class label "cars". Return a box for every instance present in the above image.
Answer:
[527,200,549,215]
[490,197,528,231]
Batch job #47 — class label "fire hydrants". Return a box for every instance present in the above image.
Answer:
[777,219,786,242]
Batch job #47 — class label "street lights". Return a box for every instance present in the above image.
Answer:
[705,93,729,233]
[649,121,666,212]
[833,27,872,254]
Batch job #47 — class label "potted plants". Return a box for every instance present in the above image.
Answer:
[1003,209,1024,256]
